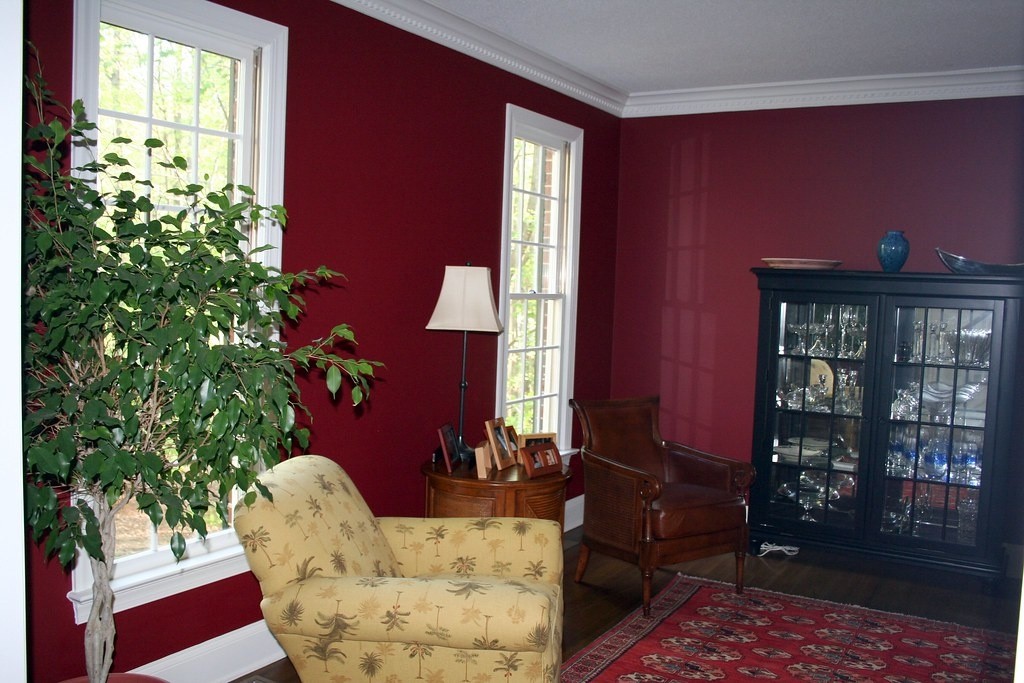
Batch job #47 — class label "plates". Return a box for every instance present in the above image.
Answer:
[762,258,841,269]
[773,448,821,460]
[789,437,837,449]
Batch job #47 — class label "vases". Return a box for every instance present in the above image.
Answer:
[877,230,909,273]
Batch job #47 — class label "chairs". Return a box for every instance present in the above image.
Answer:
[569,395,757,618]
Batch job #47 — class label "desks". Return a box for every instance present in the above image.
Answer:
[422,457,574,542]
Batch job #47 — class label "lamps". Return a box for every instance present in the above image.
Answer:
[424,261,504,461]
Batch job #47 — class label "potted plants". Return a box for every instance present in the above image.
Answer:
[22,33,390,683]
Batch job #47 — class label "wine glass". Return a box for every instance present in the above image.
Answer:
[788,304,866,359]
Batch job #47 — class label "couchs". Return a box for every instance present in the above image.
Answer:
[234,455,564,682]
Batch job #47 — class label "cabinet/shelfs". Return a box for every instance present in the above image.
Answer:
[748,267,1024,599]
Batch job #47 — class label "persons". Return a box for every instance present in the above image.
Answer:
[533,454,542,468]
[510,440,554,469]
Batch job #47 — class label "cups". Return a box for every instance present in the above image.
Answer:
[818,369,858,413]
[887,423,978,484]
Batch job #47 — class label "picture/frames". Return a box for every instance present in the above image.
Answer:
[474,417,563,480]
[437,422,463,475]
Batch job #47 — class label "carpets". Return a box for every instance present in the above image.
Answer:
[560,572,1019,683]
